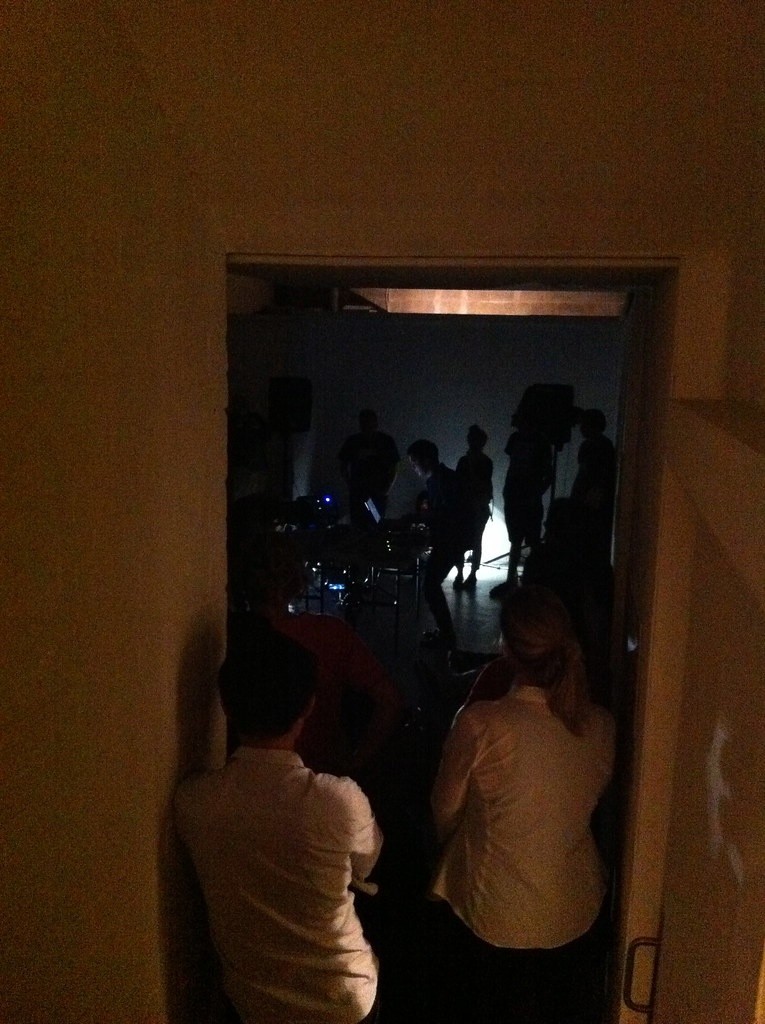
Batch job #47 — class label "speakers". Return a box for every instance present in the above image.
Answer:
[516,384,574,441]
[267,377,313,442]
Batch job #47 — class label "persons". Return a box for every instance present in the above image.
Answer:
[228,335,619,789]
[173,629,385,1024]
[426,583,617,1024]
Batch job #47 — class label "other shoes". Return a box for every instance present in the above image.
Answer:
[454,575,463,589]
[421,629,459,651]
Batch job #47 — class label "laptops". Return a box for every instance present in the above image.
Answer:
[365,497,404,536]
